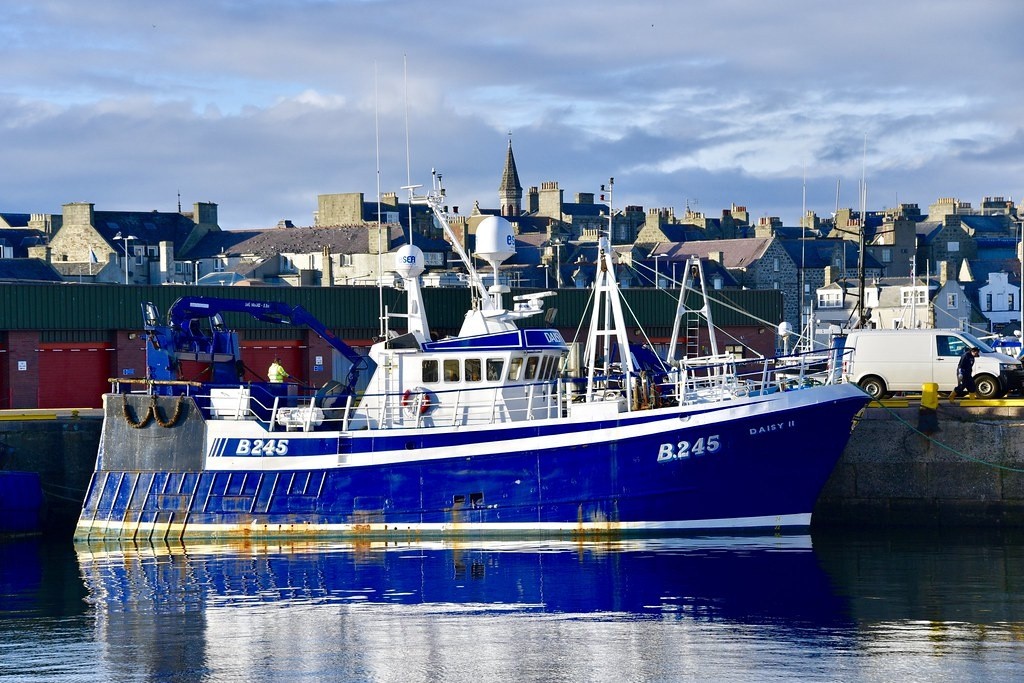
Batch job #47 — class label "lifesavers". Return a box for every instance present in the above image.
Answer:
[401,386,431,418]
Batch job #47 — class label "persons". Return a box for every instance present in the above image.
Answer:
[948,347,980,403]
[267,357,292,383]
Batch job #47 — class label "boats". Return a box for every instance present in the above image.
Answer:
[71,53,868,551]
[73,551,859,635]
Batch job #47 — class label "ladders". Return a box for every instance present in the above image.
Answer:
[687,312,698,357]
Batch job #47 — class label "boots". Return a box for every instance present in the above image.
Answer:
[949,388,957,405]
[969,392,975,400]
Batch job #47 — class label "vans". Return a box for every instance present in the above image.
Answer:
[842,328,1024,399]
